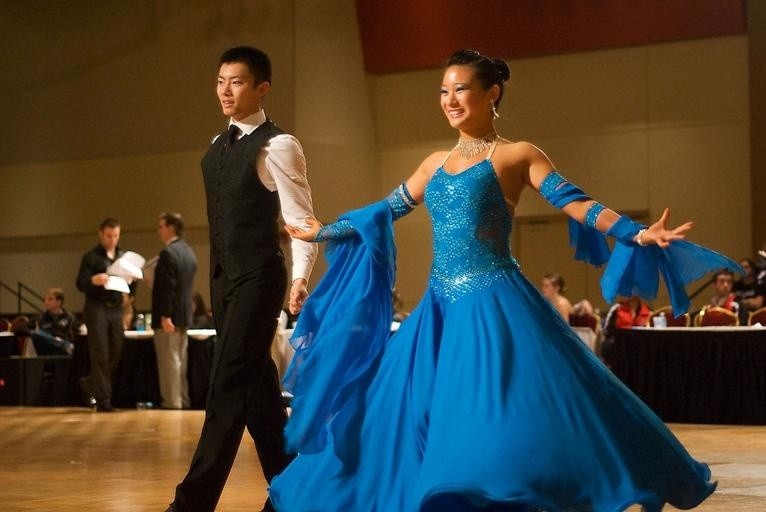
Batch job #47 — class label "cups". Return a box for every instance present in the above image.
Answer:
[653,316,667,328]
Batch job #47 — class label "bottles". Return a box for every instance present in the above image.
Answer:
[145,313,152,329]
[136,314,144,330]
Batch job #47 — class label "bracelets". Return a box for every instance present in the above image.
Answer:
[638,227,652,246]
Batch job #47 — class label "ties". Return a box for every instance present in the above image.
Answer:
[225,124,238,151]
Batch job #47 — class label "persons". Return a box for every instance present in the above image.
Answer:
[282,49,696,511]
[1,288,89,356]
[604,292,651,356]
[75,217,133,412]
[278,299,301,331]
[164,47,316,512]
[541,271,601,334]
[709,259,765,327]
[389,286,411,322]
[122,282,137,330]
[192,290,212,329]
[152,211,198,409]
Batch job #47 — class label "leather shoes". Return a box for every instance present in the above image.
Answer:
[96,399,121,412]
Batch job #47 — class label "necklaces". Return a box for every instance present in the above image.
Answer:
[457,134,496,160]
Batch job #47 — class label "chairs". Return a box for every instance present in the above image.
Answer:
[561,287,765,428]
